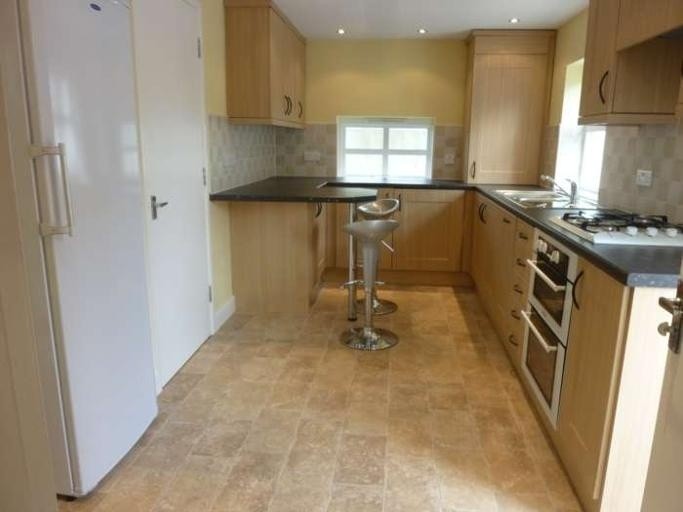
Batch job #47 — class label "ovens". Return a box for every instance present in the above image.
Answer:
[519,228,578,427]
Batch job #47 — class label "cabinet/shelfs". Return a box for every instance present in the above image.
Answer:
[229,200,329,314]
[578,0,683,124]
[464,29,557,185]
[469,191,533,367]
[222,0,306,130]
[334,190,465,285]
[554,258,629,498]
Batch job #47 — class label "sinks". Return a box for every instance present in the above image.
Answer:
[512,198,617,210]
[495,190,572,202]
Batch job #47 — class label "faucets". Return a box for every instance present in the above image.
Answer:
[539,174,578,204]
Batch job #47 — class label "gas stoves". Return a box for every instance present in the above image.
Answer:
[548,208,683,247]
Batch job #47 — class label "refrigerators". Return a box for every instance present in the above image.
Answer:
[28,0,160,503]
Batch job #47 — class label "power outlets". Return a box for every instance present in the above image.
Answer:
[637,170,652,186]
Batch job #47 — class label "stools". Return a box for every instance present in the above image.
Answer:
[340,219,403,350]
[357,198,399,316]
